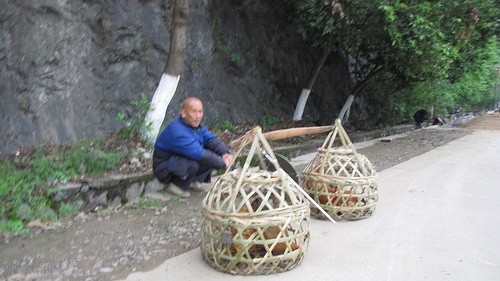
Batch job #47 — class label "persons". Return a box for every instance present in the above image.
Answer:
[151,98,234,199]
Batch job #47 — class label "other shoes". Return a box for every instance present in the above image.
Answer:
[190,179,207,192]
[169,183,190,197]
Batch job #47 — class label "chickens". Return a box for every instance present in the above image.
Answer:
[307,166,358,210]
[230,199,299,255]
